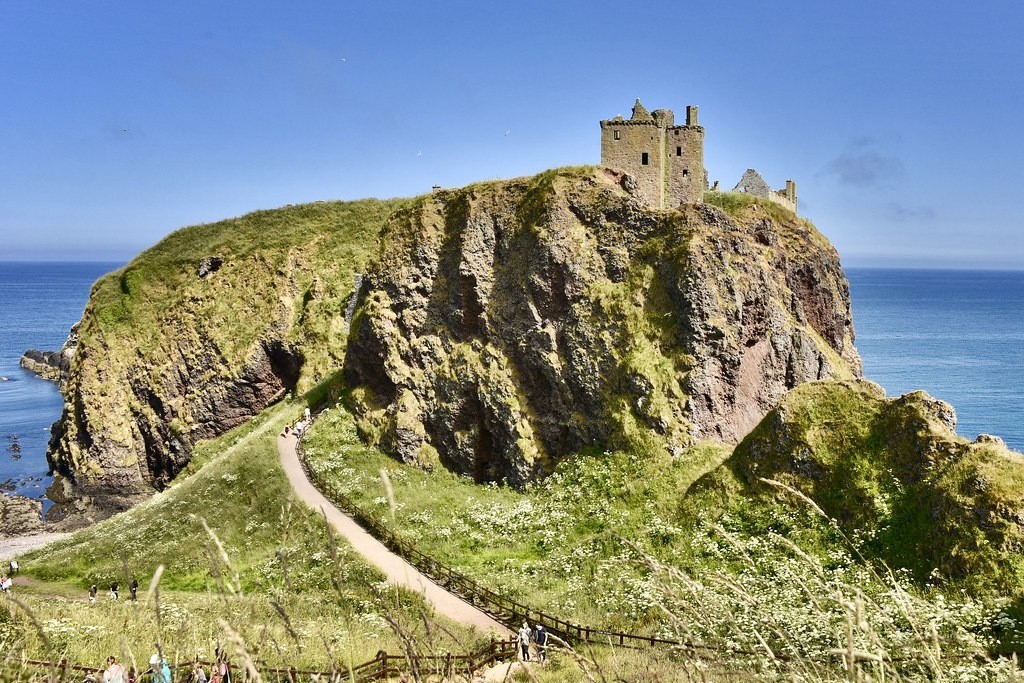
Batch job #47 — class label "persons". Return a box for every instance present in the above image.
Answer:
[295,421,303,437]
[89,584,96,603]
[130,580,138,598]
[0,574,8,592]
[516,622,531,662]
[305,406,310,421]
[284,423,289,438]
[110,582,118,599]
[93,649,230,683]
[533,622,547,662]
